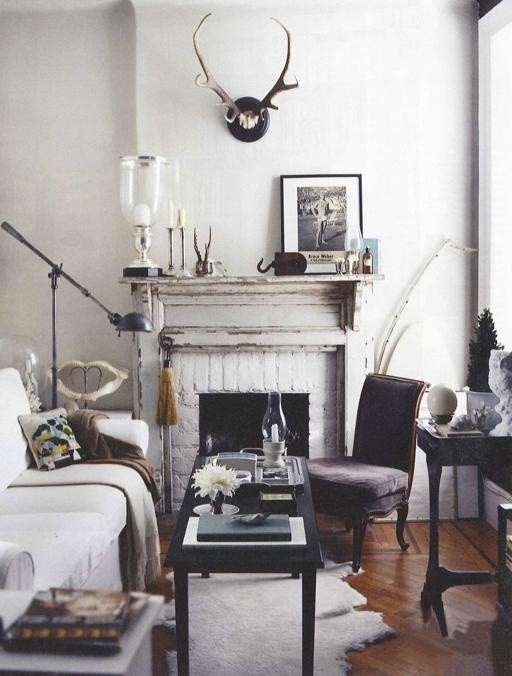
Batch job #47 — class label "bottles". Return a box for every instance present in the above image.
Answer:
[363,246,373,273]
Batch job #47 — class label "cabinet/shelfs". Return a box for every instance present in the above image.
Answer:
[0,589,166,675]
[494,501,512,630]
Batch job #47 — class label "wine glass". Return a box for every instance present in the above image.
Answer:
[345,260,354,275]
[336,261,343,274]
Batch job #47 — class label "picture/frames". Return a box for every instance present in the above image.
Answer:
[278,172,364,277]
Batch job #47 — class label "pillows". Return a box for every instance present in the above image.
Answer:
[17,405,89,471]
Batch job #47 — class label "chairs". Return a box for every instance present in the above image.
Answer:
[306,372,433,573]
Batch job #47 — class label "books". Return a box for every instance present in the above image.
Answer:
[0,584,153,656]
[197,514,291,541]
[503,535,512,576]
[434,417,483,440]
[182,516,308,551]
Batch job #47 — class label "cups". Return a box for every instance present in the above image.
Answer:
[234,469,251,485]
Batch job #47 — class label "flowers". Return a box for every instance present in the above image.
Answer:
[186,453,242,501]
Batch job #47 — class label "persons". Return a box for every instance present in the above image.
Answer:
[311,192,332,248]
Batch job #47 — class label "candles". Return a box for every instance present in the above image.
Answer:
[177,205,187,227]
[167,198,174,228]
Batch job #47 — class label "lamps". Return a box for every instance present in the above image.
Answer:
[259,389,289,470]
[0,219,155,412]
[1,332,43,410]
[115,152,172,279]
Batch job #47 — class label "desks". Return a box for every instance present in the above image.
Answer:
[161,453,326,675]
[413,412,511,639]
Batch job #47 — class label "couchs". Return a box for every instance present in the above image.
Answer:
[1,367,148,597]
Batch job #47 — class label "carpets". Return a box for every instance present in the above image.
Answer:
[154,559,399,675]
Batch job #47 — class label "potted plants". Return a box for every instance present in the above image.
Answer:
[462,306,507,432]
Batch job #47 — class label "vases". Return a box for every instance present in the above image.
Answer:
[210,491,225,515]
